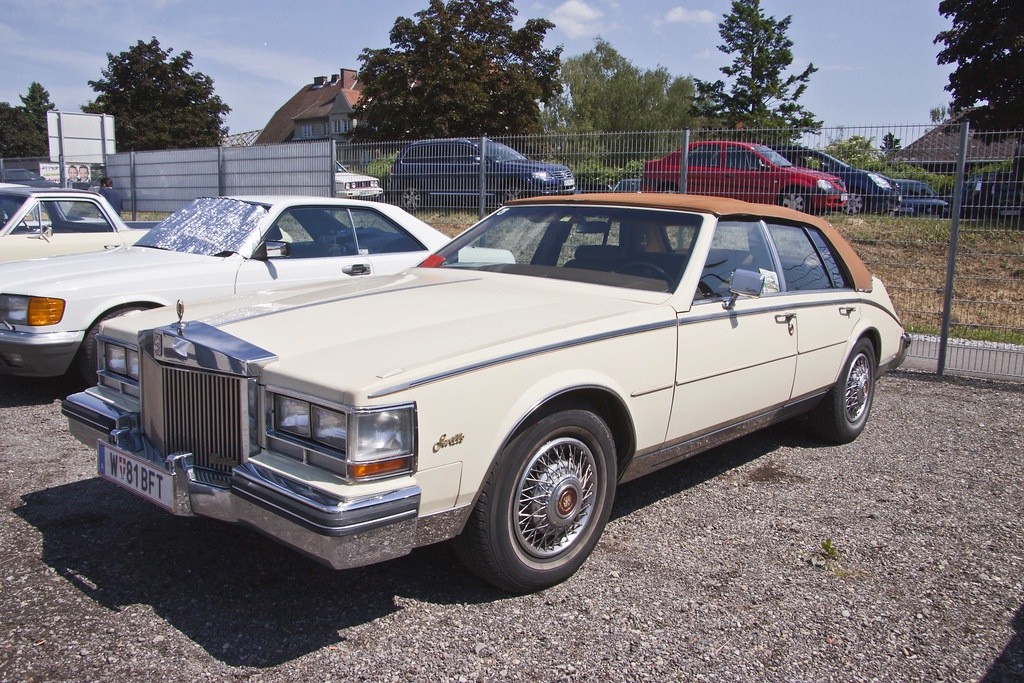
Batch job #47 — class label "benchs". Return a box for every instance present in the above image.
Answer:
[699,248,824,308]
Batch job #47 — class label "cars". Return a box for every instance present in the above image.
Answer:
[893,178,949,220]
[64,192,911,587]
[0,193,518,393]
[1,167,58,188]
[962,143,1024,214]
[769,144,892,213]
[606,180,640,193]
[640,140,852,212]
[333,160,383,199]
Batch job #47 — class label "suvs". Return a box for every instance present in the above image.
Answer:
[388,137,575,210]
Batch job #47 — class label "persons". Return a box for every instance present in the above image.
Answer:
[98,178,124,217]
[66,165,80,182]
[77,166,90,183]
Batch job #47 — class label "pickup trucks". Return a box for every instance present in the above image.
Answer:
[2,186,293,262]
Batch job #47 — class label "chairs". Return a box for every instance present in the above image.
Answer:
[563,243,623,274]
[624,251,686,289]
[267,224,283,241]
